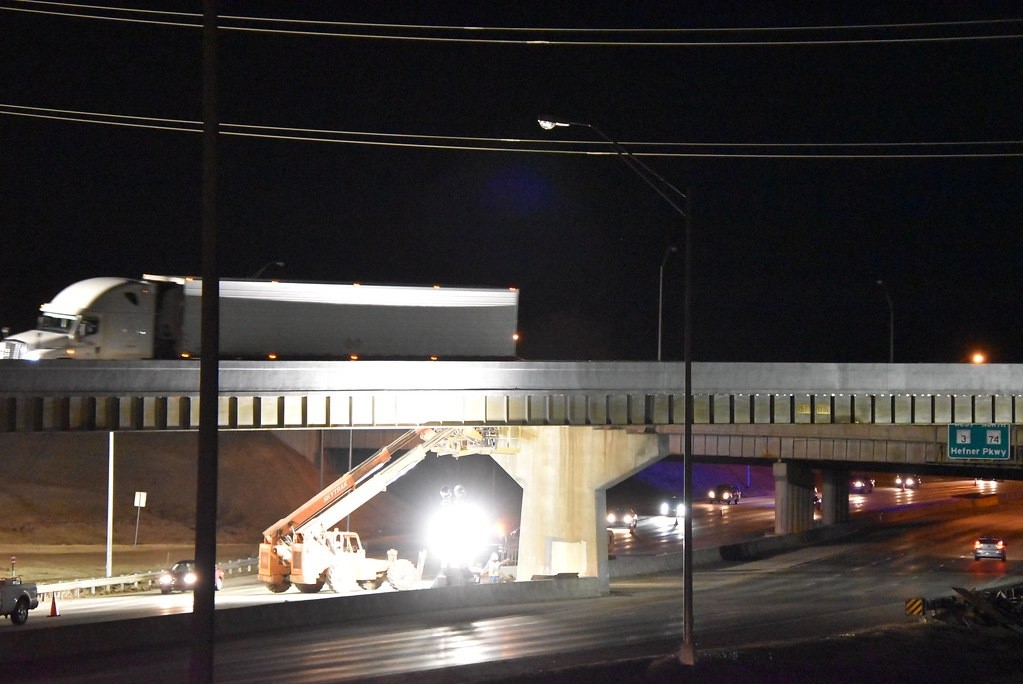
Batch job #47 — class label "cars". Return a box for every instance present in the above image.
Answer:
[850,479,875,494]
[898,474,921,488]
[974,538,1006,561]
[660,496,684,516]
[606,508,638,528]
[709,485,741,504]
[159,559,224,594]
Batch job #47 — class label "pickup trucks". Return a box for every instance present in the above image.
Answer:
[0,577,39,626]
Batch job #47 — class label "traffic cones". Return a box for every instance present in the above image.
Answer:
[47,591,61,618]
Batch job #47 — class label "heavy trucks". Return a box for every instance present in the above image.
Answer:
[0,272,518,359]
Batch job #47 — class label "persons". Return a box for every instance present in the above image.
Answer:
[488,554,500,583]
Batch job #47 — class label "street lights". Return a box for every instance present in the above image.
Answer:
[536,114,696,665]
[658,246,676,362]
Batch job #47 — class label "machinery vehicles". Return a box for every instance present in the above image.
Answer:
[259,424,500,596]
